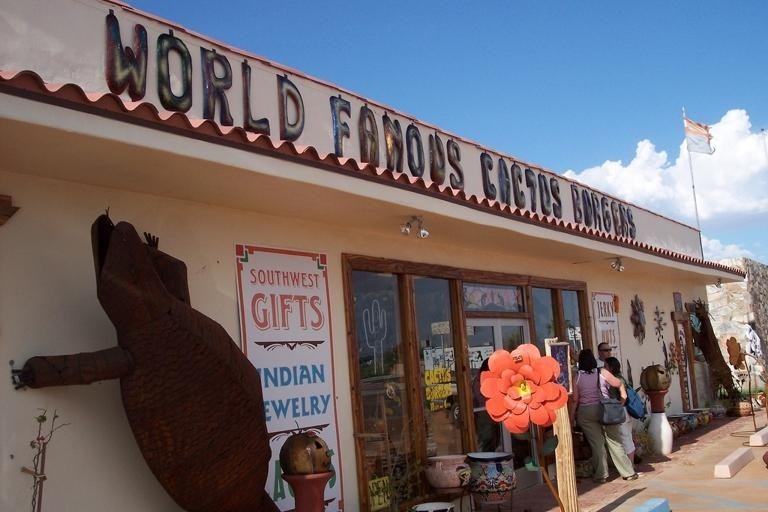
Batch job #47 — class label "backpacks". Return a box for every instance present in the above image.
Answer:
[620,378,646,418]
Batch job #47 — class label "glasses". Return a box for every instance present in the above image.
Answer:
[598,349,611,351]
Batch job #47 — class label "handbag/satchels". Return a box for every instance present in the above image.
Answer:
[599,398,625,424]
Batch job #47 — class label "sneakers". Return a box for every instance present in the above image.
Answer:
[622,473,638,480]
[592,478,606,482]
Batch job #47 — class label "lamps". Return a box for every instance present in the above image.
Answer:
[608,257,625,272]
[399,215,430,240]
[715,277,723,288]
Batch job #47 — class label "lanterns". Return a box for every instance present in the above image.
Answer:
[279,432,334,474]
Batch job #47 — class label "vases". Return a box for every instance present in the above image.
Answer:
[457,452,517,505]
[280,471,337,512]
[645,390,766,456]
[426,454,468,496]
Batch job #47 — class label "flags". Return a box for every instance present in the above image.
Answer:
[684,116,715,154]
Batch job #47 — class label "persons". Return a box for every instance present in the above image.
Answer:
[570,349,638,483]
[604,357,637,472]
[595,342,616,467]
[471,357,501,453]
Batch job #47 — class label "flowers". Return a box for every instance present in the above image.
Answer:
[480,343,569,512]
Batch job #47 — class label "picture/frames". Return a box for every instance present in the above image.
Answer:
[673,292,683,312]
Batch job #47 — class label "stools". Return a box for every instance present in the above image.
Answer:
[411,502,456,512]
